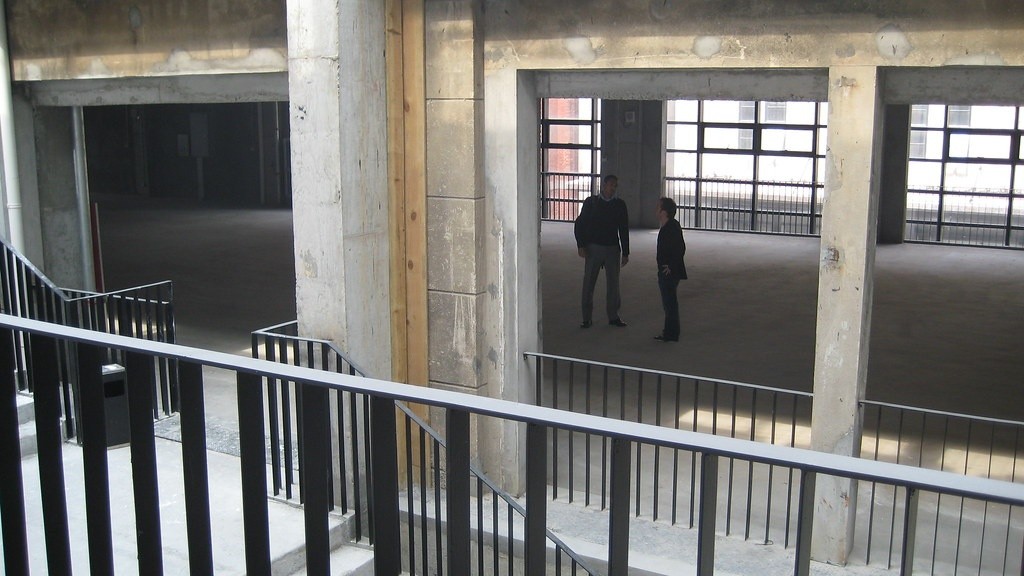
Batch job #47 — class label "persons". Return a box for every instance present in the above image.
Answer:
[654,197,687,342]
[575,175,630,328]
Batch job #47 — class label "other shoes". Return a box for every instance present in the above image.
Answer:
[653,335,667,342]
[609,319,627,327]
[581,320,592,328]
